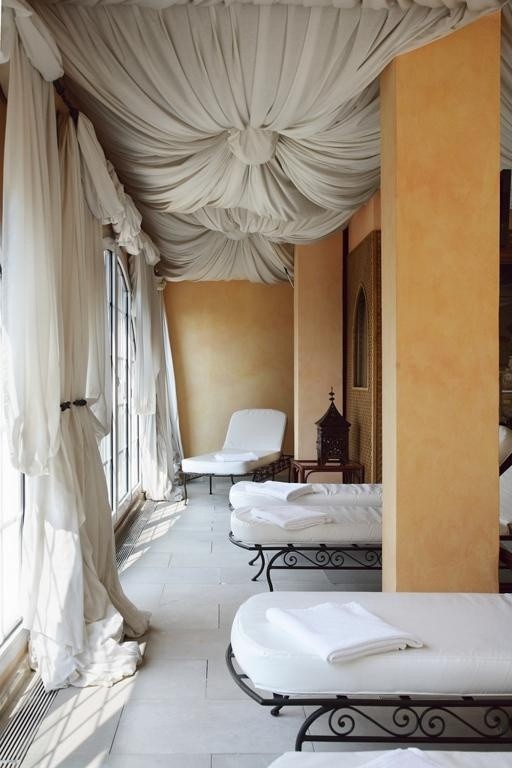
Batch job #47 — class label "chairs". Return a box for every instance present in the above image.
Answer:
[210,423,512,506]
[231,469,512,590]
[223,590,511,753]
[181,409,289,504]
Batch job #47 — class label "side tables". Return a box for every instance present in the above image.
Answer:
[294,461,365,484]
